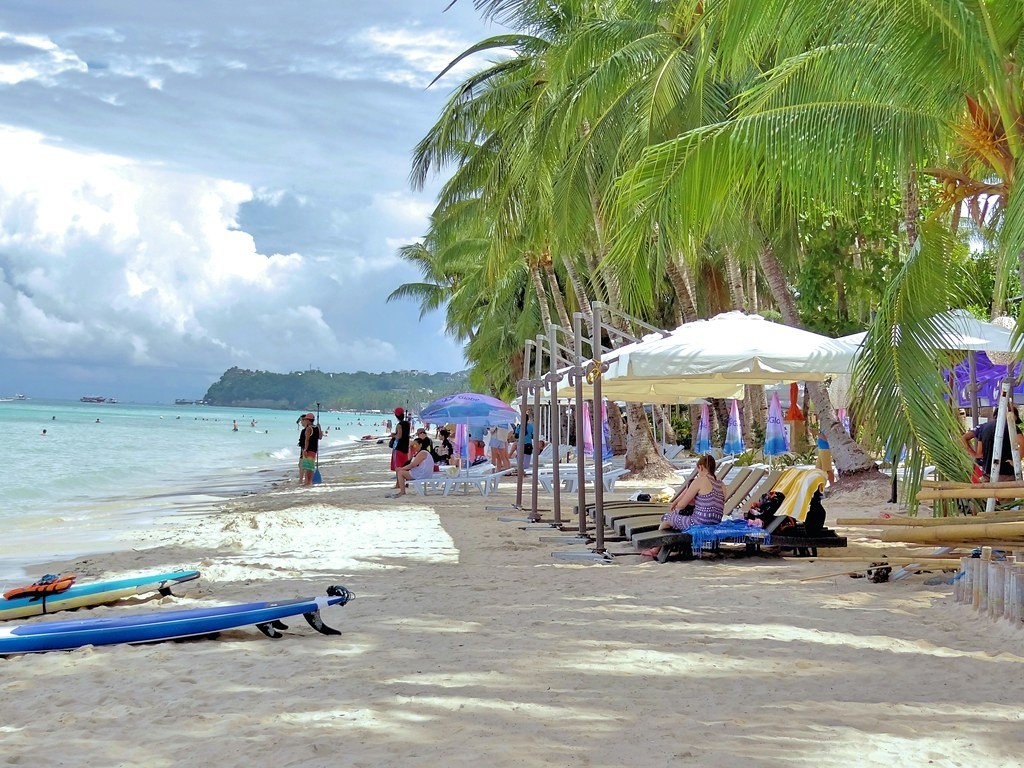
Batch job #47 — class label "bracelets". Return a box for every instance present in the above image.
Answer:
[304,448,307,451]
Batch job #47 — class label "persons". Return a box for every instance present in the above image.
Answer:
[252,419,256,426]
[811,429,834,485]
[381,410,545,496]
[303,413,322,486]
[233,420,238,432]
[670,479,698,532]
[962,405,1024,510]
[642,455,725,556]
[298,415,309,484]
[390,408,410,488]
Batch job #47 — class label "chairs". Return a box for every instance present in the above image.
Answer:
[399,431,848,558]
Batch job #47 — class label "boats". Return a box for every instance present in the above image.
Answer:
[0,397,13,403]
[107,398,117,404]
[16,394,26,400]
[80,396,107,403]
[175,398,194,405]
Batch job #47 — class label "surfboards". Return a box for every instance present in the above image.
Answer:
[0,592,355,655]
[0,570,200,620]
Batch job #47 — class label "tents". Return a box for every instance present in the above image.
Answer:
[511,302,1024,558]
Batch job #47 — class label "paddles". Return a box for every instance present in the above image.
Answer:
[312,402,321,483]
[405,399,409,421]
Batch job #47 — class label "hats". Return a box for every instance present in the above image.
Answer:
[395,407,404,415]
[416,429,425,435]
[301,415,306,418]
[303,413,315,419]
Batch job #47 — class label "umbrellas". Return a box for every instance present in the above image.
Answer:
[614,401,654,415]
[763,391,789,474]
[454,424,468,468]
[574,401,593,456]
[593,400,613,461]
[694,401,712,457]
[419,394,519,494]
[724,399,745,466]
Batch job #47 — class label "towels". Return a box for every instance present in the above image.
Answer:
[770,466,828,525]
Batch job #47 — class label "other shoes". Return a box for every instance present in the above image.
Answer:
[642,549,658,557]
[385,494,397,499]
[480,456,487,461]
[478,441,485,448]
[471,457,483,466]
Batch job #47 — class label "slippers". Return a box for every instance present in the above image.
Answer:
[595,550,615,562]
